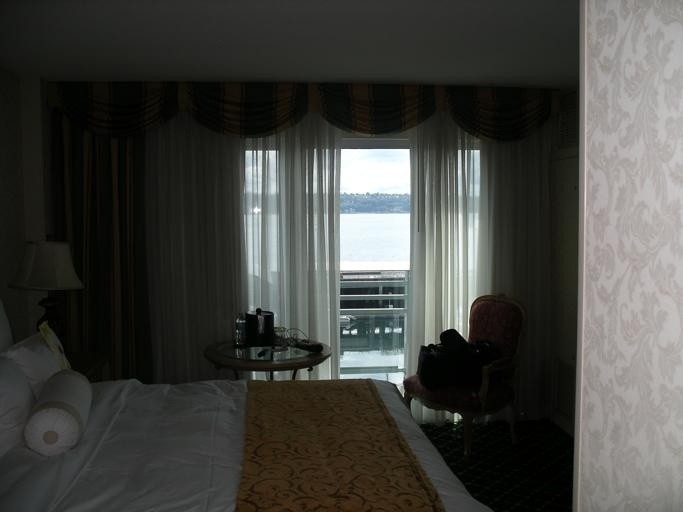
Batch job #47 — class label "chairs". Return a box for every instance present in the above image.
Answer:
[402,295,528,444]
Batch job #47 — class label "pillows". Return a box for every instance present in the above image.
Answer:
[1,321,71,400]
[0,354,36,458]
[23,370,93,457]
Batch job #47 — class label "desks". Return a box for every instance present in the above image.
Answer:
[198,340,332,381]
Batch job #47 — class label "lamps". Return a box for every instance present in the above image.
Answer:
[8,241,86,355]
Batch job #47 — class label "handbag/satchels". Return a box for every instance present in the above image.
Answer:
[463,341,497,370]
[419,344,459,391]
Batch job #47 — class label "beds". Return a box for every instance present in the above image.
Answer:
[0,298,488,512]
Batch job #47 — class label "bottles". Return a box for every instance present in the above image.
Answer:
[236,312,246,345]
